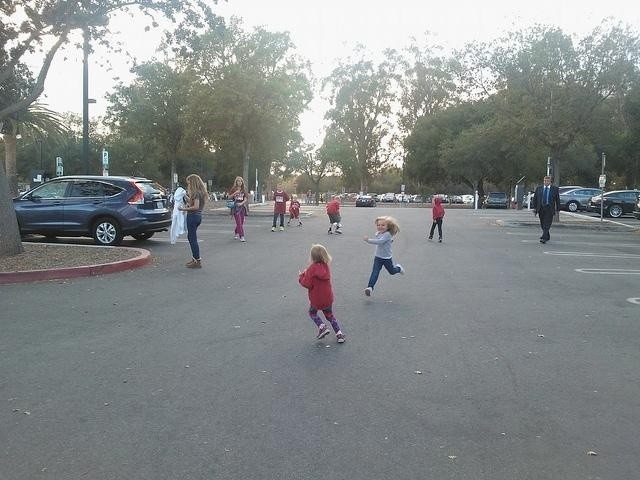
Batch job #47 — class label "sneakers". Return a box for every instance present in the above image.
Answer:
[233,232,238,239]
[364,287,373,296]
[287,221,290,225]
[396,264,405,274]
[328,231,333,234]
[335,229,343,234]
[299,221,302,225]
[316,325,330,339]
[272,226,276,231]
[429,236,433,239]
[440,239,442,242]
[337,334,346,343]
[279,226,284,231]
[239,236,246,241]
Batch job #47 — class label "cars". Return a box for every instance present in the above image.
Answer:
[632,194,640,220]
[559,188,606,212]
[12,175,174,245]
[381,192,475,203]
[511,186,582,209]
[483,192,508,209]
[328,192,381,203]
[588,189,639,217]
[356,196,376,207]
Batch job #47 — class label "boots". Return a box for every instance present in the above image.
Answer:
[186,257,202,268]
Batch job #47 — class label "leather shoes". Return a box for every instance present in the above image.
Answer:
[540,239,548,243]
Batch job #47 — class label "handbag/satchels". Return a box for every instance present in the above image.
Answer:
[225,199,234,208]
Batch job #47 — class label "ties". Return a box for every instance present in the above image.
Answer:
[543,186,547,204]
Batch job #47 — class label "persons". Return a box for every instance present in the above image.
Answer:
[306,189,312,206]
[479,195,491,210]
[297,244,346,343]
[221,190,228,200]
[227,175,250,242]
[270,182,290,232]
[319,192,323,204]
[286,193,304,226]
[532,175,561,243]
[362,215,405,298]
[426,197,446,242]
[325,194,344,235]
[177,173,209,268]
